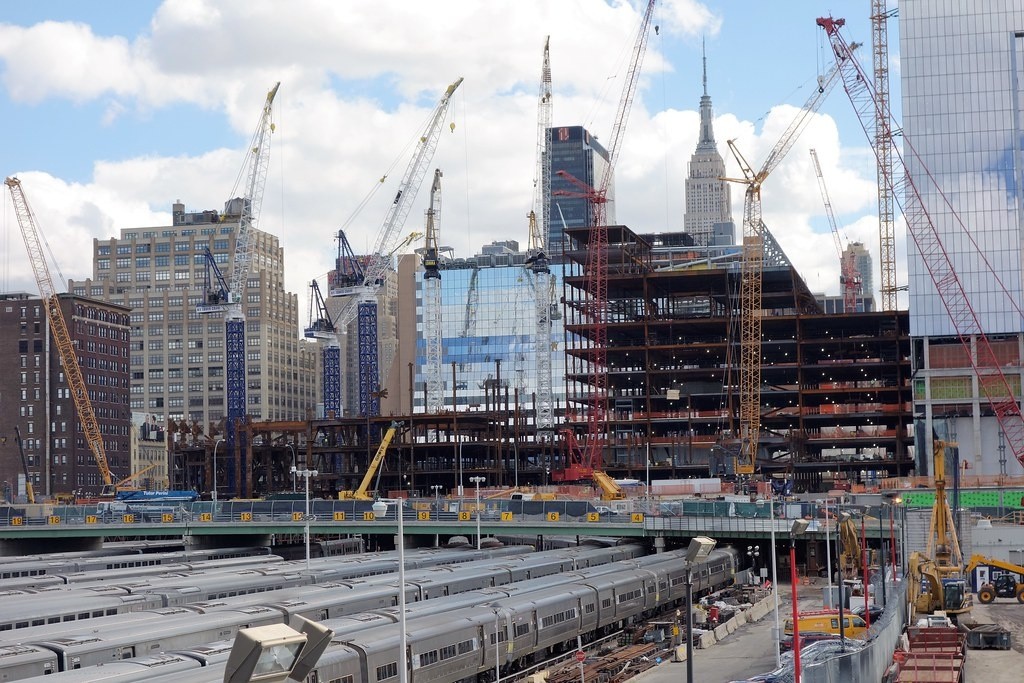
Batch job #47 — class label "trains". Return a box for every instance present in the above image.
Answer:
[1,538,741,683]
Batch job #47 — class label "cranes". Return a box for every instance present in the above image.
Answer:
[3,0,1023,523]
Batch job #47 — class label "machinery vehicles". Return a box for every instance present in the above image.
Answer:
[962,553,1024,605]
[905,436,973,627]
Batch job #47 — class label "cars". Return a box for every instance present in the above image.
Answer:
[850,604,885,624]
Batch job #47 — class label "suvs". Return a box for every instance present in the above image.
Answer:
[780,630,833,656]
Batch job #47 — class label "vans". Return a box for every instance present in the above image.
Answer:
[784,614,871,641]
[793,634,842,656]
